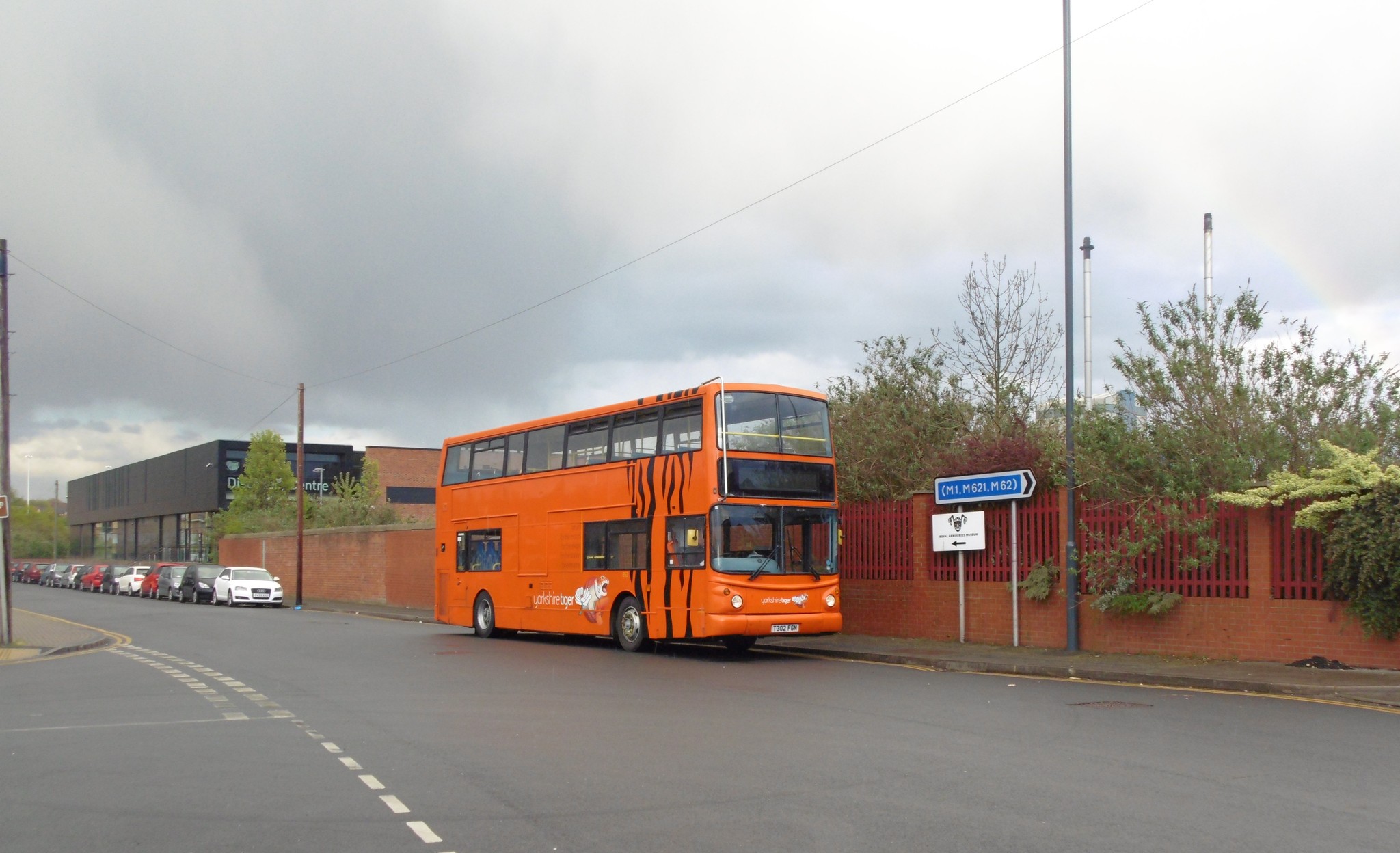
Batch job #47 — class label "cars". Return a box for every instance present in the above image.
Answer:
[211,566,284,608]
[9,561,110,593]
[115,566,151,596]
[155,566,189,602]
[98,565,135,595]
[178,564,227,605]
[139,562,185,599]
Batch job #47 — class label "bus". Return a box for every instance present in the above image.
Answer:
[432,375,846,655]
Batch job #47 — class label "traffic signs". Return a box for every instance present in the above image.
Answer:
[931,510,986,552]
[933,466,1039,506]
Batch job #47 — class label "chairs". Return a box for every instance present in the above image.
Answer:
[237,572,267,581]
[459,540,503,570]
[454,443,718,485]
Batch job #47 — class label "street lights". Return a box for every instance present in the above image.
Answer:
[25,455,33,515]
[312,467,325,509]
